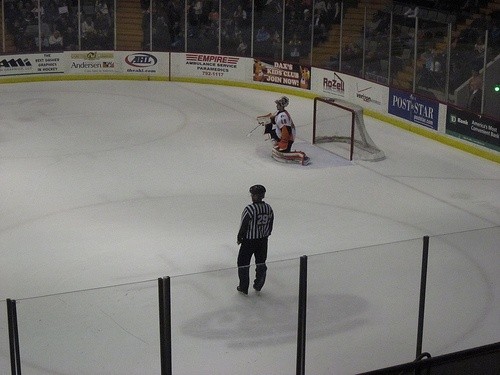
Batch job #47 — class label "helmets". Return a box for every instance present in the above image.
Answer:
[275,96,289,107]
[250,185,266,194]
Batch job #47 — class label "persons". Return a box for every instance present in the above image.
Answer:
[265,96,307,165]
[236,184,274,295]
[0,0,500,115]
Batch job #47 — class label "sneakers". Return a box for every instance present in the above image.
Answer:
[237,286,248,297]
[253,284,260,295]
[302,155,311,166]
